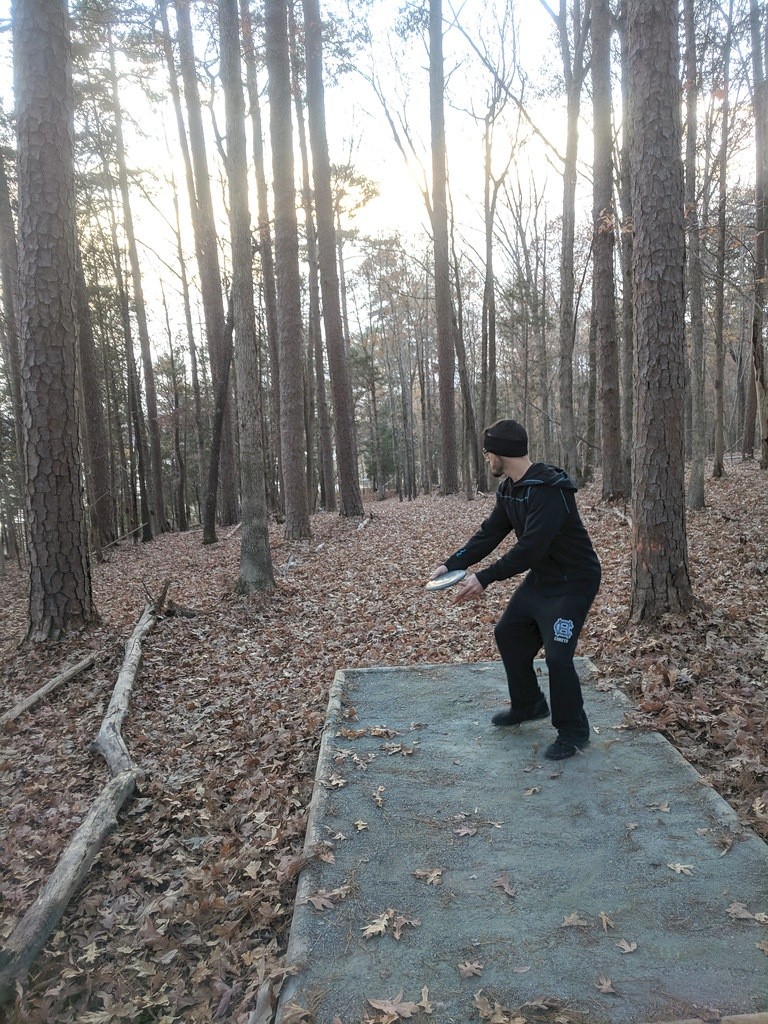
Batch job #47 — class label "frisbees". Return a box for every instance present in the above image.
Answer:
[425,569,467,591]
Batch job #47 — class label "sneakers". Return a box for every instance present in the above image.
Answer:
[492,698,548,725]
[545,735,590,762]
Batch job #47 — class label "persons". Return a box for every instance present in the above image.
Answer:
[429,420,602,761]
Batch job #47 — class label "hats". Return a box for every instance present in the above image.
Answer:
[484,419,527,457]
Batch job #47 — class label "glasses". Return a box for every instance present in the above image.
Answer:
[482,450,489,459]
